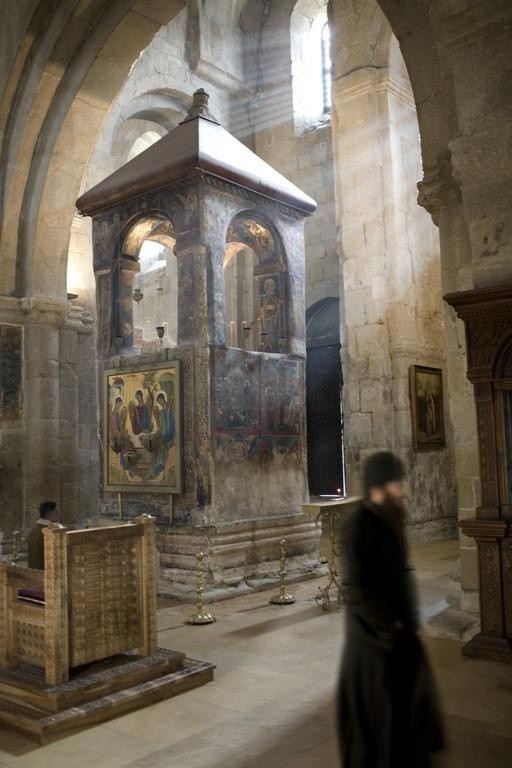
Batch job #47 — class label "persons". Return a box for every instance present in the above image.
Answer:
[144,391,154,433]
[128,400,141,435]
[424,382,437,437]
[143,393,175,471]
[336,448,451,768]
[25,501,65,569]
[135,389,148,433]
[110,396,138,477]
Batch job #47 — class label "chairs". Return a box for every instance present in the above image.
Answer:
[0,512,163,689]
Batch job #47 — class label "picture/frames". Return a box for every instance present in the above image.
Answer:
[102,359,184,495]
[408,363,448,450]
